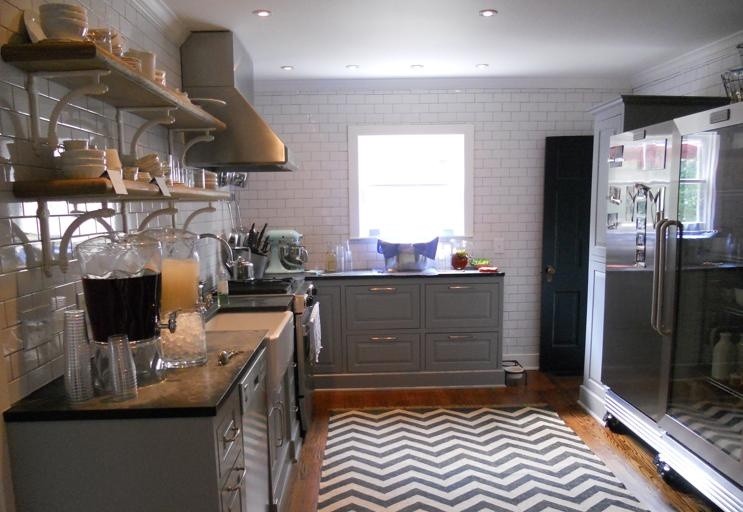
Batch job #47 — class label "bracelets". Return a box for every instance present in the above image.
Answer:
[39,3,88,40]
[59,149,106,179]
[504,365,524,379]
[122,152,164,183]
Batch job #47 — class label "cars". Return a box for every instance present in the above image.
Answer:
[58,140,87,152]
[166,154,196,189]
[61,309,93,404]
[107,333,139,402]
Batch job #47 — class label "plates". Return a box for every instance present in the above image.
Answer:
[0,40,231,276]
[6,389,247,512]
[313,278,502,373]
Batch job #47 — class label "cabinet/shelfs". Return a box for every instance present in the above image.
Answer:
[214,265,229,307]
[711,332,735,382]
[326,240,352,272]
[294,296,304,314]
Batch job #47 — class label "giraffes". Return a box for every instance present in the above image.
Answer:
[210,276,298,295]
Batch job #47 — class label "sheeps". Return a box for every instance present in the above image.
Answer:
[198,233,233,263]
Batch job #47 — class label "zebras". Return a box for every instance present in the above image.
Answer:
[295,301,321,433]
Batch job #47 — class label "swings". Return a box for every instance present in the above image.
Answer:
[265,229,307,274]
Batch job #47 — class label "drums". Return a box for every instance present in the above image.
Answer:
[247,222,270,256]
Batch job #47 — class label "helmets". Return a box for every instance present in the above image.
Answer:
[22,7,41,43]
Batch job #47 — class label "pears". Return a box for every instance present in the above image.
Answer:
[216,265,230,309]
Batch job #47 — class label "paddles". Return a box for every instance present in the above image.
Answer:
[315,403,653,512]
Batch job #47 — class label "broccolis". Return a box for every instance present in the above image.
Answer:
[597,97,742,494]
